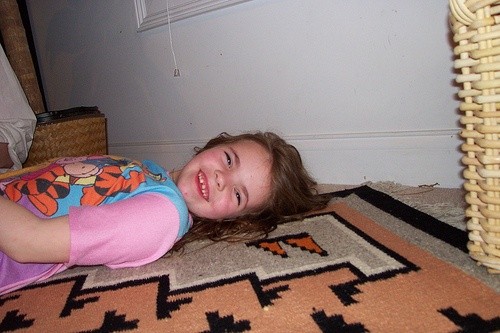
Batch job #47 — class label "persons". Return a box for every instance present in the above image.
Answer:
[0,131,334,296]
[0,43,37,175]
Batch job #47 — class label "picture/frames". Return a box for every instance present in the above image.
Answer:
[134,0,251,32]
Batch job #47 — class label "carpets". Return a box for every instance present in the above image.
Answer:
[0,185,500,333]
